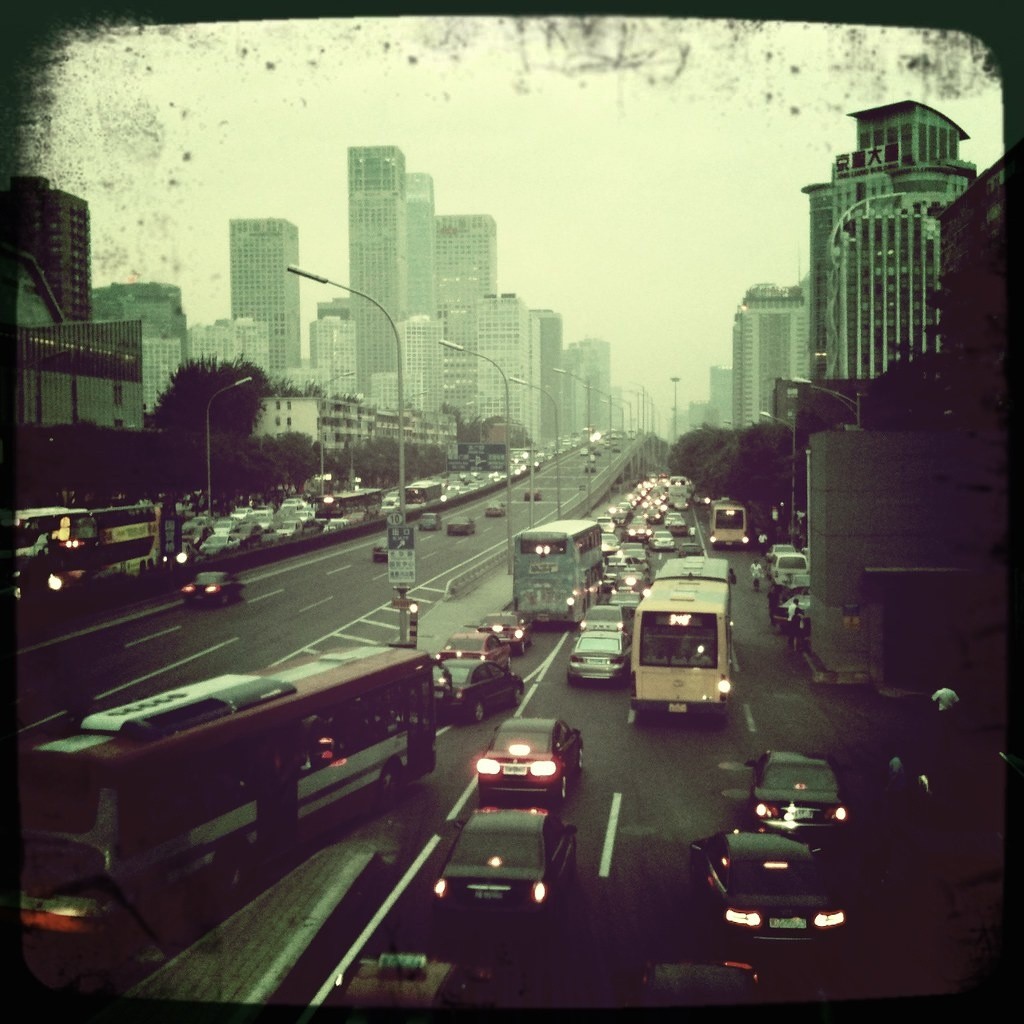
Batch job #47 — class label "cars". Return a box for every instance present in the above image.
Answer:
[335,472,849,1006]
[168,431,638,611]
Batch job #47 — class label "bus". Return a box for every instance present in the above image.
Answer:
[632,555,733,722]
[669,477,690,511]
[513,519,606,624]
[21,645,437,982]
[711,499,748,549]
[15,501,168,603]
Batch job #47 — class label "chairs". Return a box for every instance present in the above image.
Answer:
[669,655,688,667]
[648,654,668,666]
[689,656,714,668]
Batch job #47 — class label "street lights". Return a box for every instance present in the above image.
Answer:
[206,376,253,517]
[438,337,514,576]
[445,401,474,471]
[509,377,561,520]
[317,371,357,496]
[553,368,591,517]
[286,263,410,528]
[579,383,612,503]
[601,386,653,490]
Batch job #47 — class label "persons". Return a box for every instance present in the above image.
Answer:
[928,680,959,741]
[758,531,767,557]
[767,584,811,654]
[750,560,764,587]
[688,525,696,544]
